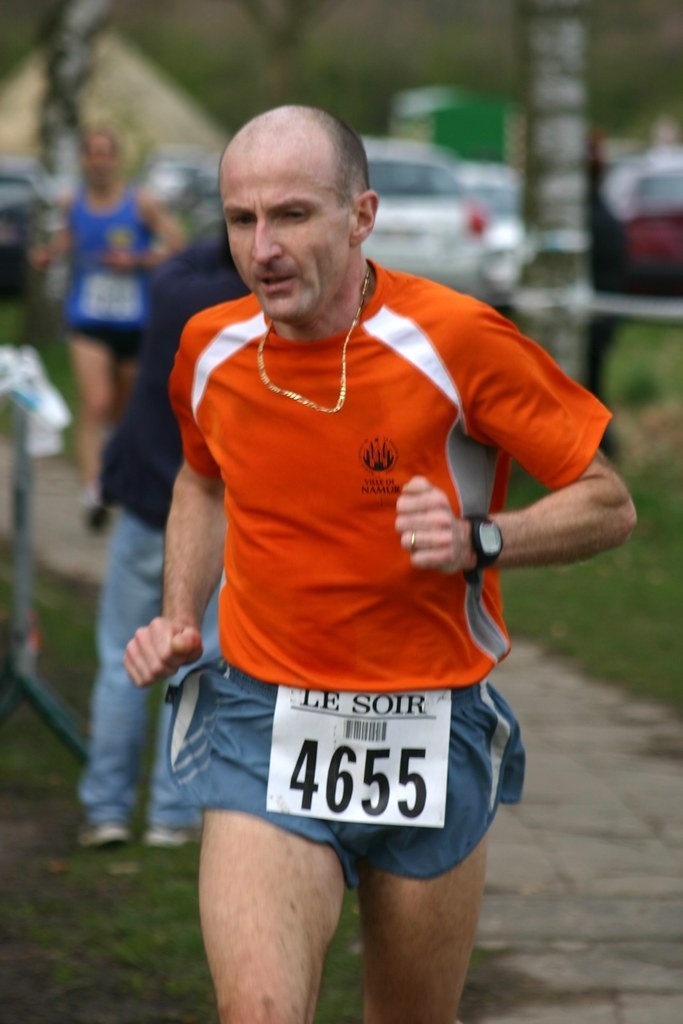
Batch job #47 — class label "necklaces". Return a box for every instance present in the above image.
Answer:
[257,270,372,413]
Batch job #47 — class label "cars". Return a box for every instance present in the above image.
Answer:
[0,152,59,305]
[594,142,683,298]
[355,133,525,312]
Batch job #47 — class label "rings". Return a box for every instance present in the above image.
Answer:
[410,528,415,549]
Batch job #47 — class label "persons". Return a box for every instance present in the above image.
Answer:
[29,102,636,1024]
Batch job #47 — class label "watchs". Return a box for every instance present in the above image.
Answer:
[462,514,503,571]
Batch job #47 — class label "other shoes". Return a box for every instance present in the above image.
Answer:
[75,819,131,847]
[146,824,189,848]
